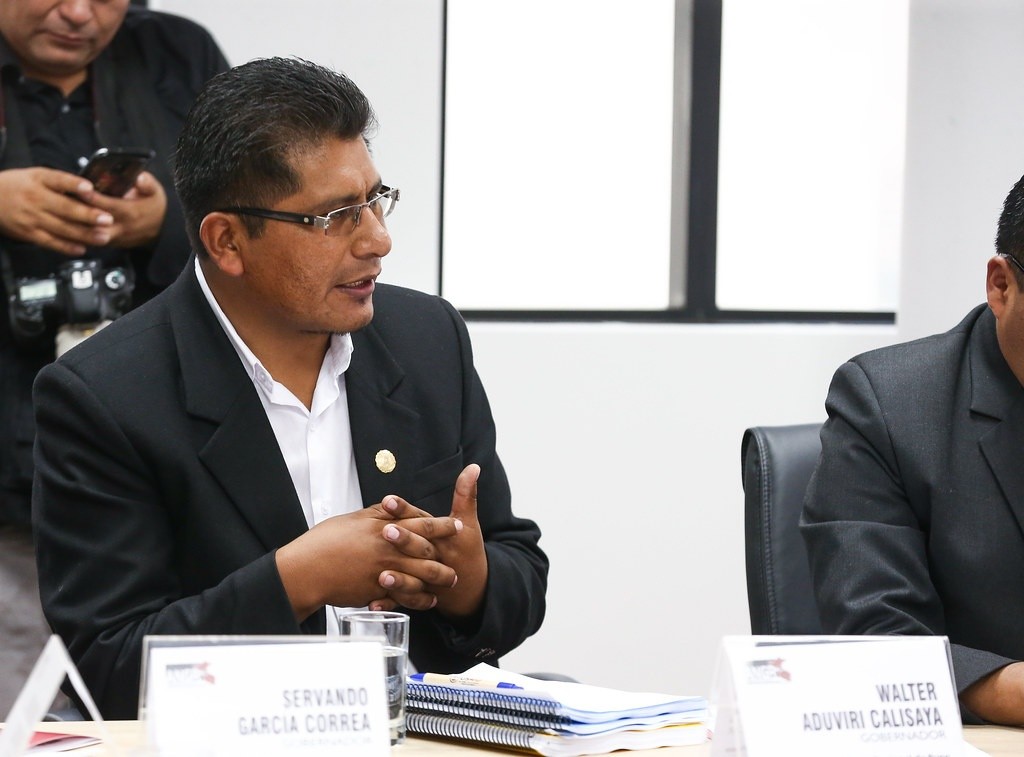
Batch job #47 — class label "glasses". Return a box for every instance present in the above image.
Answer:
[213,185,400,238]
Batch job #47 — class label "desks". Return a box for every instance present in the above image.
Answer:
[1,706,1024,756]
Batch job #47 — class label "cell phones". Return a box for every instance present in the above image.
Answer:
[65,147,154,207]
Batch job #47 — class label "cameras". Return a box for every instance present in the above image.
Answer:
[6,253,144,360]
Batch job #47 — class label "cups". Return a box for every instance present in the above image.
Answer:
[339,611,411,746]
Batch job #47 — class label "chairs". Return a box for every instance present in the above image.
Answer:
[738,420,832,636]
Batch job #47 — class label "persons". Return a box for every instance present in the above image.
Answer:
[792,172,1024,729]
[0,0,251,729]
[29,51,554,722]
